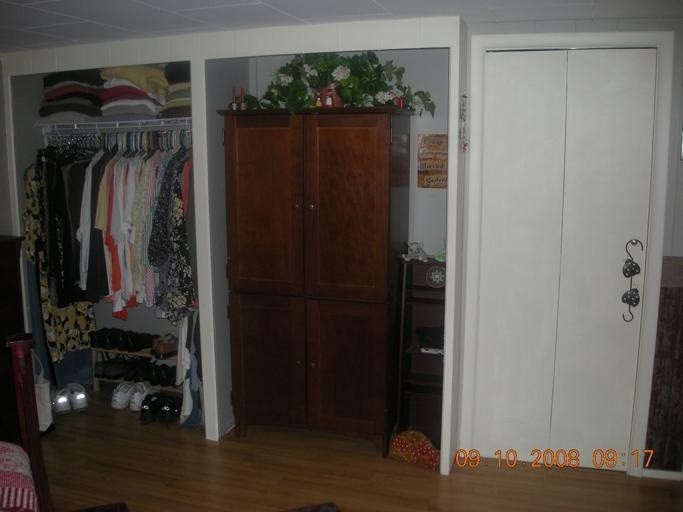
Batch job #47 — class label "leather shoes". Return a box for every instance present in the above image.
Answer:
[103,327,123,350]
[151,333,174,356]
[150,376,159,386]
[118,329,138,352]
[89,327,109,348]
[156,337,177,360]
[128,332,151,353]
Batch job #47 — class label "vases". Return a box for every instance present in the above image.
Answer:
[312,81,344,110]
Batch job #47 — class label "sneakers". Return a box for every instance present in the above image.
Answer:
[111,381,136,411]
[53,387,72,417]
[95,351,183,389]
[129,380,153,413]
[66,382,89,412]
[140,391,164,425]
[156,396,182,423]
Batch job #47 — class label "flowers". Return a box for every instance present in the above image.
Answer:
[228,56,435,118]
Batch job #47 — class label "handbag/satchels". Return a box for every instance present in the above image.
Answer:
[29,349,53,432]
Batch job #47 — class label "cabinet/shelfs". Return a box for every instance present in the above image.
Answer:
[89,345,183,393]
[216,106,396,458]
[0,234,26,447]
[397,259,446,451]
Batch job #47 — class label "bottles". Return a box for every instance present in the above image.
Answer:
[315,89,334,109]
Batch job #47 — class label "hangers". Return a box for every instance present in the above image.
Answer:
[45,127,191,153]
